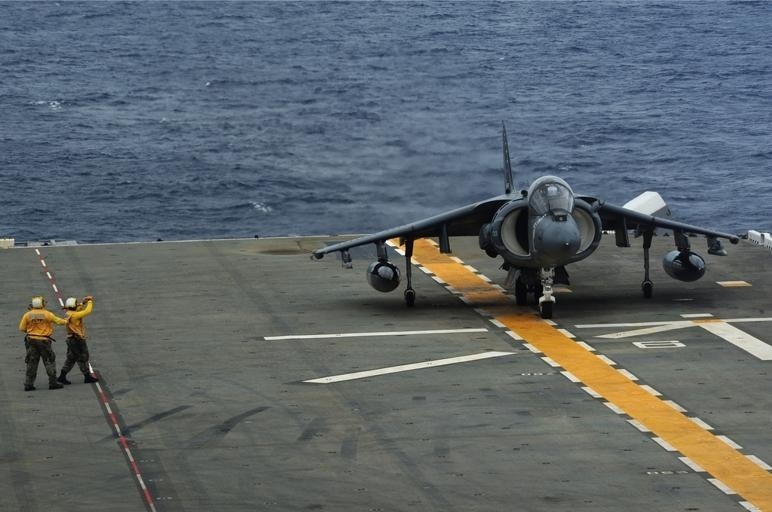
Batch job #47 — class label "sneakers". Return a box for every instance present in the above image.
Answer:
[49,383,63,389]
[24,386,36,390]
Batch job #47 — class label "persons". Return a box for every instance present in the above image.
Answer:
[56,296,100,384]
[19,296,73,391]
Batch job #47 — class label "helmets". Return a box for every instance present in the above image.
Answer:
[64,297,77,310]
[31,295,45,309]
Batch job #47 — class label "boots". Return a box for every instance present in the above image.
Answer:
[57,370,72,385]
[84,372,98,383]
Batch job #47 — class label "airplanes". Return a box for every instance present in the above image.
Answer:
[310,117,740,321]
